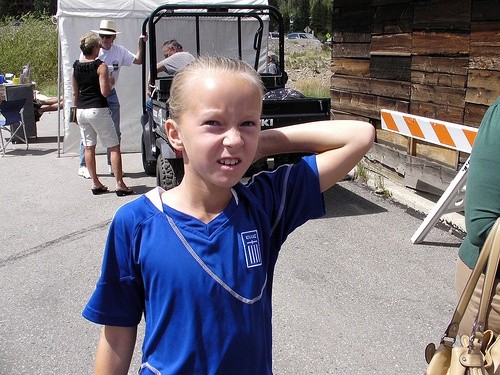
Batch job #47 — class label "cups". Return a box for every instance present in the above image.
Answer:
[108,66,114,78]
[24,72,30,84]
[0,75,4,84]
[13,78,19,85]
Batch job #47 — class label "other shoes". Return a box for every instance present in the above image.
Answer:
[78,166,91,179]
[108,166,124,176]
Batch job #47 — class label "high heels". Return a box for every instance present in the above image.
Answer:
[91,185,108,194]
[115,187,136,196]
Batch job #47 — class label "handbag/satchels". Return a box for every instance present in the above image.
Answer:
[425,218,500,375]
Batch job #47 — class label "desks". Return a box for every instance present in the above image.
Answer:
[0,81,36,99]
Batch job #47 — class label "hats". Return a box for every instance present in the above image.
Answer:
[90,20,122,35]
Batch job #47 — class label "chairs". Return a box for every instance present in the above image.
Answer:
[0,99,28,157]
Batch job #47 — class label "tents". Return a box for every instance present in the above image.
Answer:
[56,0,270,159]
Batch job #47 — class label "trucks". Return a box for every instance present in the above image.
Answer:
[141,3,331,191]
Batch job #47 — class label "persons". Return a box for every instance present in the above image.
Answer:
[81,56,375,375]
[32,94,64,118]
[454,95,500,348]
[78,18,149,179]
[70,33,134,197]
[264,51,278,74]
[145,40,196,100]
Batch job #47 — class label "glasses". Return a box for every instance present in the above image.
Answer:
[99,34,112,38]
